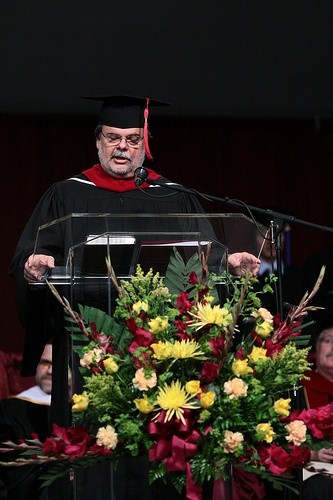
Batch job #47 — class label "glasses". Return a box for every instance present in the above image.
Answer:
[101,131,144,147]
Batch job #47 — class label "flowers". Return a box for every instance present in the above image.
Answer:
[0,247,332,500]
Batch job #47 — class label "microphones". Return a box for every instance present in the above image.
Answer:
[133,166,149,186]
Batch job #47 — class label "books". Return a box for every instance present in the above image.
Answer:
[81,234,136,276]
[132,241,212,277]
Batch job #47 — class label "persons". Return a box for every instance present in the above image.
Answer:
[289,315,333,500]
[7,95,261,500]
[0,339,72,500]
[245,206,304,320]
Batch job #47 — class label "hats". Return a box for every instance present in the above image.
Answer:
[79,93,171,162]
